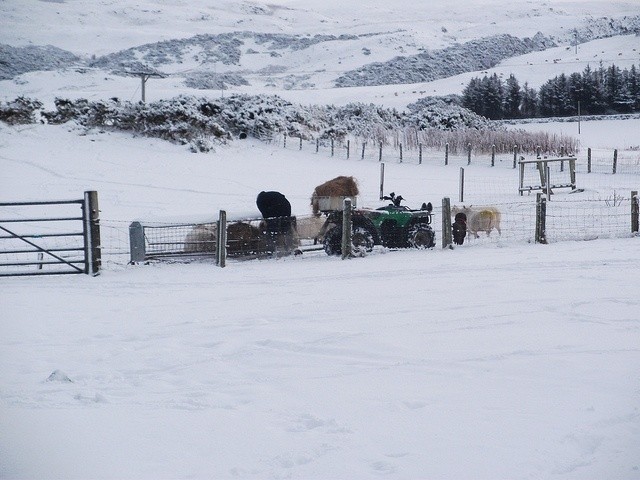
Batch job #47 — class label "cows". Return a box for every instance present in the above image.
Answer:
[183,222,217,252]
[310,176,359,218]
[451,204,501,236]
[459,204,495,239]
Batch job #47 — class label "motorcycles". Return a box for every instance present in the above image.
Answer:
[352,191,435,253]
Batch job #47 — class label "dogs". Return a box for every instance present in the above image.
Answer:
[452,212,467,247]
